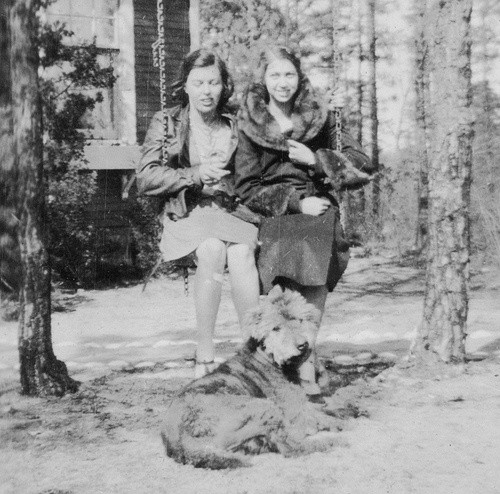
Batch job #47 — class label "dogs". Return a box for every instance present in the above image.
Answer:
[162,287,354,470]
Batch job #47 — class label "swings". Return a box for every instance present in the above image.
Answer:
[156,0,352,271]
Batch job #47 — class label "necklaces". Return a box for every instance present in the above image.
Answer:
[192,118,225,156]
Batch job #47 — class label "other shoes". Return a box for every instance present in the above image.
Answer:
[194,349,216,380]
[289,343,321,395]
[298,347,330,389]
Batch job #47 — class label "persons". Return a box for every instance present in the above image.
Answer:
[137,49,375,396]
